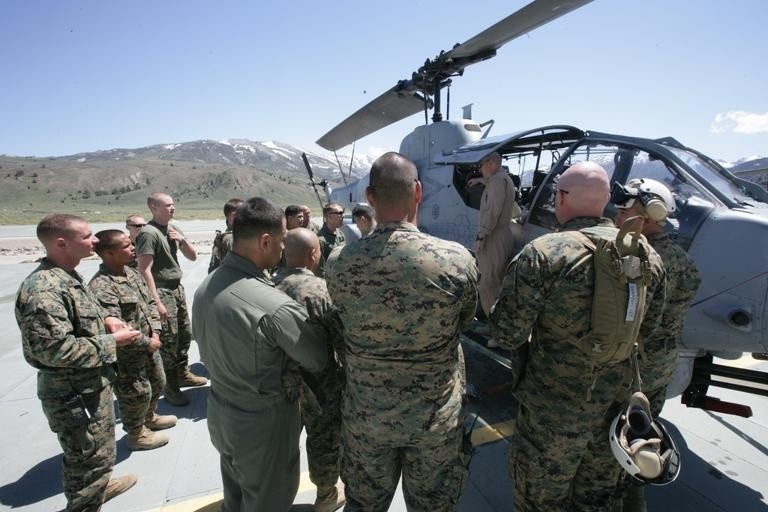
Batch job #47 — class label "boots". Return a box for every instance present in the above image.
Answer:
[315,488,347,512]
[144,413,178,431]
[103,476,137,502]
[163,385,192,407]
[488,339,500,349]
[125,432,172,450]
[178,374,208,387]
[472,326,491,336]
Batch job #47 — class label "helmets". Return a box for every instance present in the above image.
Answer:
[611,177,676,224]
[609,393,681,487]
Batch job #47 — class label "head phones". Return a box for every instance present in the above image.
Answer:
[643,200,670,223]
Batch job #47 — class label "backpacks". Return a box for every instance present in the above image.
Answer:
[540,217,659,401]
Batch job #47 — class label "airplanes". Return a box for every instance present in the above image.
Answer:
[301,1,768,418]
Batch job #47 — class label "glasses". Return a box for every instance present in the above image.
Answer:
[553,187,571,196]
[610,183,666,204]
[328,211,345,215]
[128,222,147,228]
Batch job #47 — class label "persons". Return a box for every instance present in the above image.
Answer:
[124,213,151,268]
[16,213,137,512]
[490,162,667,512]
[353,203,377,238]
[88,228,178,450]
[273,206,304,270]
[209,197,245,272]
[299,205,320,235]
[134,192,207,407]
[475,152,515,348]
[269,228,345,512]
[191,198,329,512]
[604,178,701,512]
[317,203,346,278]
[326,152,476,512]
